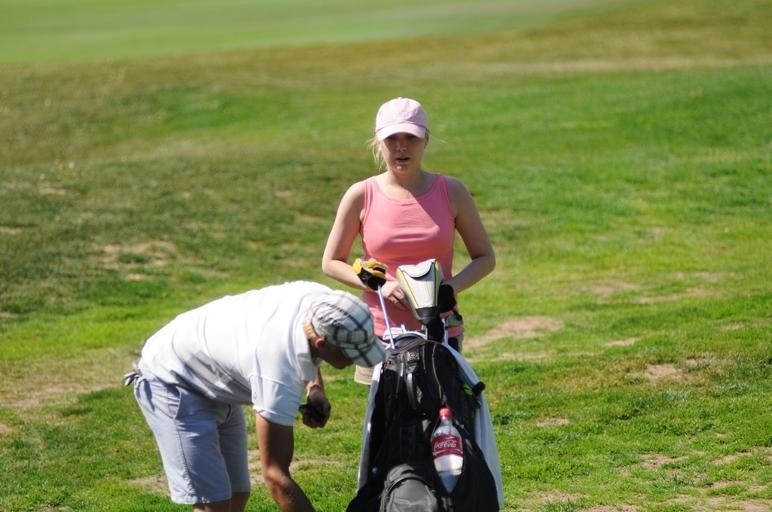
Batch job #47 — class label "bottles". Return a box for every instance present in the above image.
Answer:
[430,407,464,494]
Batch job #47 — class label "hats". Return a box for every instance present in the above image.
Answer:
[375,96,427,141]
[307,289,386,367]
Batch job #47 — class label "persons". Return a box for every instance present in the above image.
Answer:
[320,95,497,385]
[122,278,385,511]
